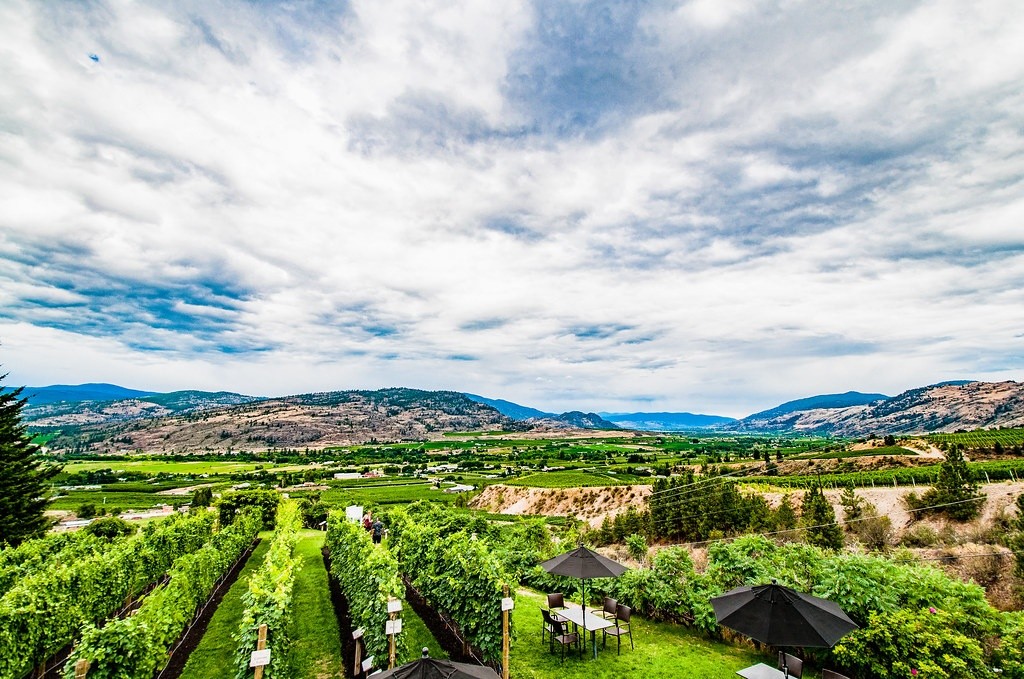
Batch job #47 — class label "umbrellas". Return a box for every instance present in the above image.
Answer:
[365,646,502,679]
[709,578,860,679]
[540,543,631,649]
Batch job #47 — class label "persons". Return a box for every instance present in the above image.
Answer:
[363,509,383,545]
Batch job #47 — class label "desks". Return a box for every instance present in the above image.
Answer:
[556,605,615,659]
[736,662,800,679]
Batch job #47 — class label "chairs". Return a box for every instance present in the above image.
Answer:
[547,614,582,662]
[604,603,634,655]
[540,608,570,650]
[822,668,851,679]
[777,651,803,679]
[588,597,619,641]
[546,592,568,633]
